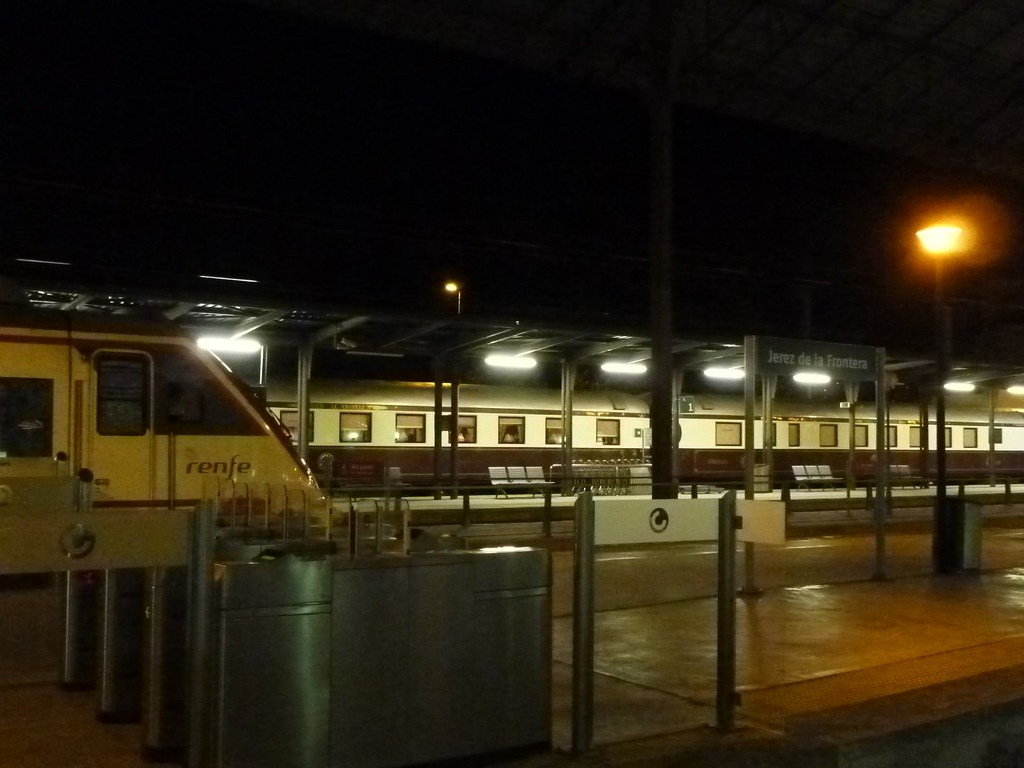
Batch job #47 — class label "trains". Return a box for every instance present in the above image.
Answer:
[264,396,1024,490]
[1,301,338,589]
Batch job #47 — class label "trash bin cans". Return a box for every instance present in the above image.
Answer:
[932,494,984,571]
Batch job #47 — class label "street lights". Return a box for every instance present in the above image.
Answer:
[916,219,965,575]
[444,281,463,501]
